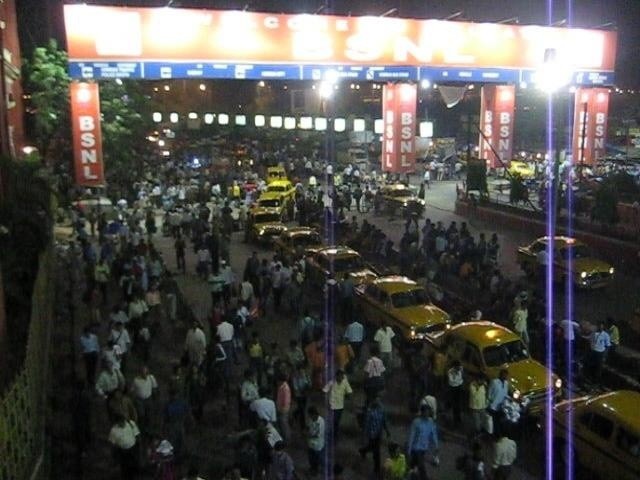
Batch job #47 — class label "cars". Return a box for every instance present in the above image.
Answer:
[537,389,639,479]
[515,234,617,293]
[376,184,426,212]
[247,167,378,295]
[347,273,454,348]
[420,319,566,420]
